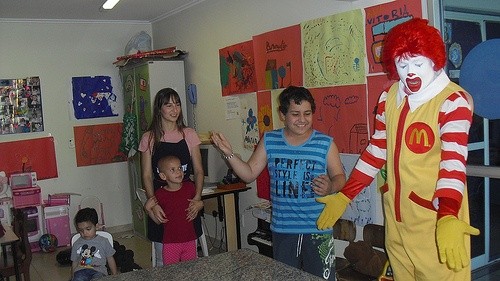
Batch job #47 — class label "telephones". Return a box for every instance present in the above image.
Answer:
[187,81,198,106]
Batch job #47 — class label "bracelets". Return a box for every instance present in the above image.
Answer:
[223,152,234,159]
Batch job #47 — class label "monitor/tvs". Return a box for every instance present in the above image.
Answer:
[10,171,37,189]
[189,144,231,184]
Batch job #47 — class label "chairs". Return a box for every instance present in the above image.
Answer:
[79,196,106,233]
[0,208,32,281]
[333,219,393,281]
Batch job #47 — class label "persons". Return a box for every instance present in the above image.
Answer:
[315,18,480,281]
[1,80,43,135]
[211,85,347,281]
[138,87,204,268]
[71,207,118,281]
[144,155,205,267]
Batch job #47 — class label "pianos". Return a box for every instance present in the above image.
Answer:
[248,205,273,259]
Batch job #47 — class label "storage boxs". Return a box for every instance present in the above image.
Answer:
[13,187,42,209]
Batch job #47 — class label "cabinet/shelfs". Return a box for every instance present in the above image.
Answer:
[121,60,188,239]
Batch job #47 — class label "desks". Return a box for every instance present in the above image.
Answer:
[90,248,328,281]
[0,217,20,281]
[193,181,251,249]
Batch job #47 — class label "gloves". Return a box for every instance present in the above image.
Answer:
[316,192,352,230]
[435,215,480,272]
